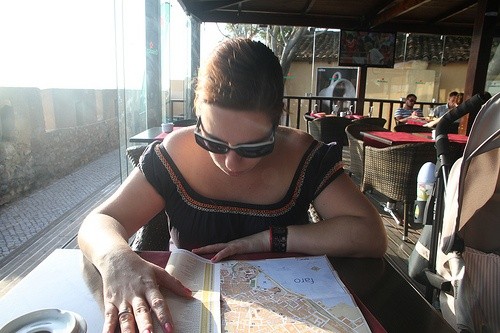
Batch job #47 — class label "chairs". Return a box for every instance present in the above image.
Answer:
[344,123,393,185]
[351,117,387,128]
[309,115,349,148]
[393,124,433,134]
[131,208,170,250]
[124,144,147,166]
[359,141,464,242]
[173,119,197,127]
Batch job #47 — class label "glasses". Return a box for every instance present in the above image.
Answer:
[193,116,276,158]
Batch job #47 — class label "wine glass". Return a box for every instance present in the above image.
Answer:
[347,105,353,114]
[368,106,373,119]
[330,105,338,115]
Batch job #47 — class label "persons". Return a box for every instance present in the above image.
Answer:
[429,91,464,122]
[77,37,388,333]
[394,94,424,134]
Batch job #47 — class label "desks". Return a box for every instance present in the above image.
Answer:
[0,249,460,333]
[128,126,189,143]
[359,130,469,226]
[305,114,367,135]
[404,117,459,135]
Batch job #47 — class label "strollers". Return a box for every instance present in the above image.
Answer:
[414,92,500,333]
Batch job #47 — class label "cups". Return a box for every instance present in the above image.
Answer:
[161,123,173,132]
[319,72,355,114]
[340,112,346,117]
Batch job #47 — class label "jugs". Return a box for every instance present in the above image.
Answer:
[428,107,434,119]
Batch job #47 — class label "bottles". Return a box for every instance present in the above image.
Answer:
[432,130,436,140]
[314,103,318,113]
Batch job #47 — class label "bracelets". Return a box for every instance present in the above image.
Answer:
[270,226,288,253]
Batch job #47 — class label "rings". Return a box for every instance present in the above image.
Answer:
[117,311,132,320]
